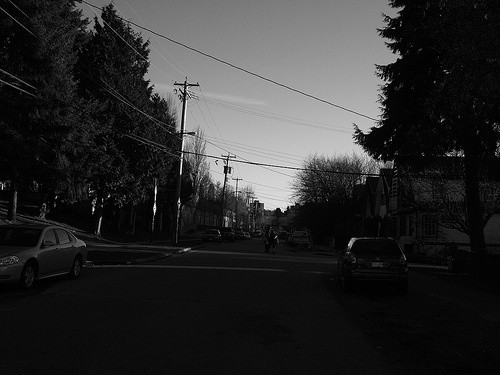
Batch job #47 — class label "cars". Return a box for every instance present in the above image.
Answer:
[235,224,292,253]
[287,230,312,249]
[202,229,221,242]
[337,236,409,296]
[0,223,87,290]
[220,227,236,241]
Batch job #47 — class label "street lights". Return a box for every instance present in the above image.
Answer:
[150,132,196,245]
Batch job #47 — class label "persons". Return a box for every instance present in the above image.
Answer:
[261,226,279,250]
